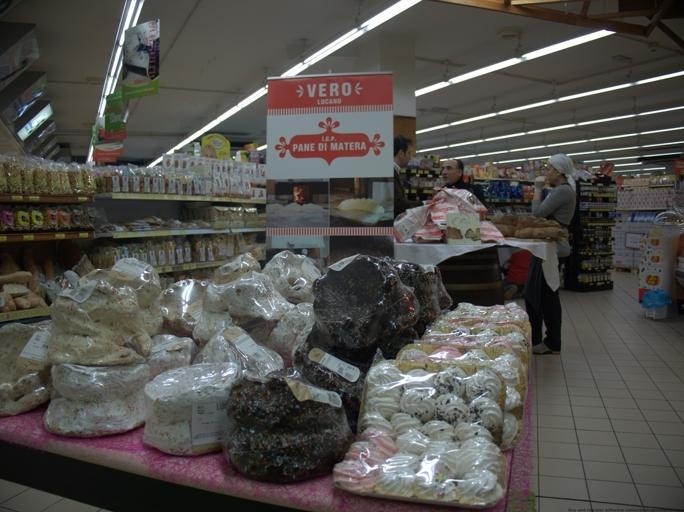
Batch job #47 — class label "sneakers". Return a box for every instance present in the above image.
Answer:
[532,342,561,355]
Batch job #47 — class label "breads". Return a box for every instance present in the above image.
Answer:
[490,212,569,239]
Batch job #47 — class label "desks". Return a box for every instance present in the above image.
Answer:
[1,362,533,512]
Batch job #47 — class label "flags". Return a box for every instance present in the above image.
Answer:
[262,72,395,266]
[92,18,161,162]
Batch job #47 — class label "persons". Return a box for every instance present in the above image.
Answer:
[438,158,486,206]
[394,134,434,220]
[523,153,578,354]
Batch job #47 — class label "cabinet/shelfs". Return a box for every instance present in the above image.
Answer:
[0,178,673,322]
